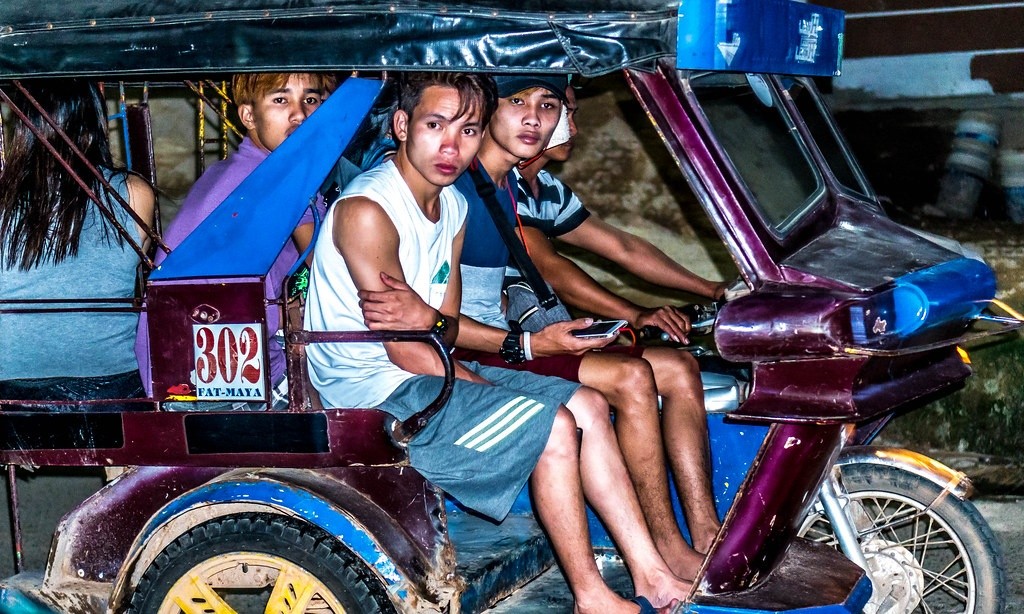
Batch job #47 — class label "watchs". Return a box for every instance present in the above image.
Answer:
[497,318,527,364]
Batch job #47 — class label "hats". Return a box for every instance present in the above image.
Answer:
[490,71,568,100]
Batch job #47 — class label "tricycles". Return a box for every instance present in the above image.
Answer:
[0,0,1024,614]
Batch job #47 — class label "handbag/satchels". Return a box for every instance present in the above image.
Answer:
[503,278,571,334]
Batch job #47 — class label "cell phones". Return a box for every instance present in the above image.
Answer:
[572,320,627,338]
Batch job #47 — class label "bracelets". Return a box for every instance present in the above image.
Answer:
[432,308,449,337]
[523,332,534,360]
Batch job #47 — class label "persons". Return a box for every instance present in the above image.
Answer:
[134,71,337,412]
[505,73,737,345]
[304,71,693,614]
[447,71,720,582]
[0,78,156,484]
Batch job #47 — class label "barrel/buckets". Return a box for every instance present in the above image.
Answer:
[936,109,1024,226]
